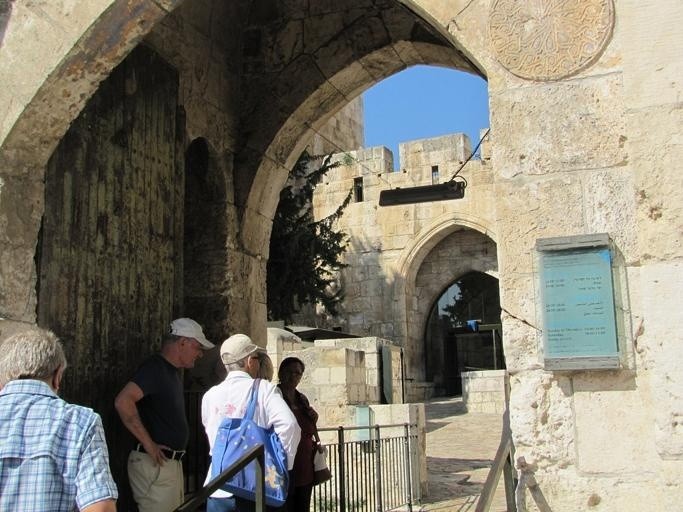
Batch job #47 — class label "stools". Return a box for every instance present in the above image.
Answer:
[132,444,182,461]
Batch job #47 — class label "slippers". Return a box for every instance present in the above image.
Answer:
[211,379,289,507]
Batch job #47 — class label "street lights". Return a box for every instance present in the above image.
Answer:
[312,415,331,485]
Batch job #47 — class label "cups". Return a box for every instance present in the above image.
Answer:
[252,356,263,361]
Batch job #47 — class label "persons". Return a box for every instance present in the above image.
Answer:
[201,334,319,511]
[0,326,119,512]
[114,316,215,512]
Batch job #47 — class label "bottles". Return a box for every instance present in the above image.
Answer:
[220,334,267,364]
[169,318,216,350]
